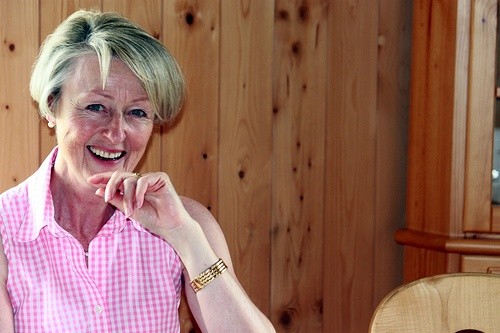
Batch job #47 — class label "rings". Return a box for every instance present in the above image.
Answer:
[132,172,141,178]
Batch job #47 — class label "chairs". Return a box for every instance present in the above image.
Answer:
[367,271,500,333]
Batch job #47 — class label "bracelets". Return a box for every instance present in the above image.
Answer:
[188,258,228,293]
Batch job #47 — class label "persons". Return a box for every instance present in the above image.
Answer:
[0,8,276,333]
